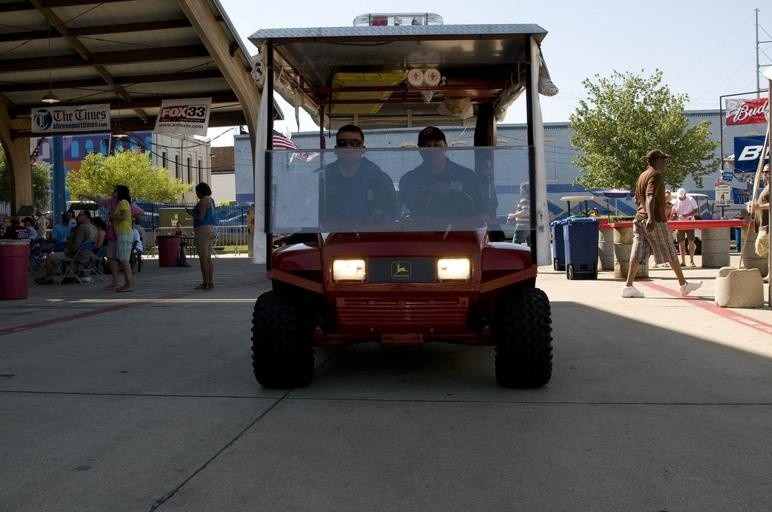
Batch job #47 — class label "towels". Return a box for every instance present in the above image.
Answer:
[1,238,33,301]
[550,220,564,271]
[157,236,182,266]
[561,215,600,280]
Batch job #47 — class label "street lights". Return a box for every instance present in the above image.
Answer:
[107,224,116,241]
[755,225,769,257]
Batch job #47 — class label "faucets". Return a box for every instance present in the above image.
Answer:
[195,283,213,289]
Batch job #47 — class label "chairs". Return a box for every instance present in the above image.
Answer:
[35,276,54,285]
[680,281,702,296]
[621,286,644,298]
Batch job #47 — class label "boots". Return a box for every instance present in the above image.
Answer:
[243,13,551,394]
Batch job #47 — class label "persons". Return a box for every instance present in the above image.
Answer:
[670,187,699,267]
[651,189,674,269]
[746,163,770,283]
[0,184,143,293]
[399,127,488,221]
[186,182,217,290]
[508,182,531,246]
[620,149,703,299]
[317,123,397,223]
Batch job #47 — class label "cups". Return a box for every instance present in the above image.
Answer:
[337,139,362,147]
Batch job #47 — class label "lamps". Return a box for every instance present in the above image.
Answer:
[29,237,109,284]
[210,234,219,257]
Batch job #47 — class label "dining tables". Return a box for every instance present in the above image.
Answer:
[613,216,635,243]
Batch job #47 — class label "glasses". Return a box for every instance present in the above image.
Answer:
[40,24,60,104]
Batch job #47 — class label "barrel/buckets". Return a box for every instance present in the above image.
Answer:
[116,286,135,292]
[104,284,119,289]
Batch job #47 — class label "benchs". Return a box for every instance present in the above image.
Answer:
[273,129,298,149]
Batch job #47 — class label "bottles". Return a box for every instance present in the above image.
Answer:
[677,188,685,197]
[418,127,445,146]
[647,149,671,158]
